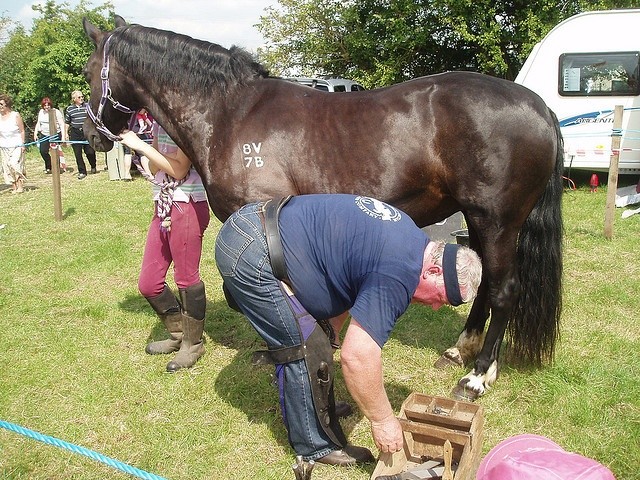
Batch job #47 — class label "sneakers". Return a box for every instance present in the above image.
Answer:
[91,168,96,174]
[77,173,86,179]
[12,185,17,195]
[16,182,24,193]
[47,168,52,174]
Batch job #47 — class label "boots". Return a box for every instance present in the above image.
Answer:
[145,283,183,355]
[167,281,206,372]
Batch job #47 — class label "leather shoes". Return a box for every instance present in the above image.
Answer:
[303,445,372,468]
[334,401,352,418]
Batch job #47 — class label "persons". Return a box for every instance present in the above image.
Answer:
[214,193,483,468]
[34,97,65,174]
[0,94,27,195]
[123,125,155,181]
[64,90,96,180]
[132,109,152,134]
[120,120,210,372]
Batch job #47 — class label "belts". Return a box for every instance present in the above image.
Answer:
[258,201,265,235]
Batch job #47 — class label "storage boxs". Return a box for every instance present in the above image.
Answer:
[369,391,486,480]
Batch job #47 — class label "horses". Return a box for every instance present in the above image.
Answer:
[83,14,562,403]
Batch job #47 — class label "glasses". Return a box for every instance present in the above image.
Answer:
[0,103,6,105]
[78,96,83,99]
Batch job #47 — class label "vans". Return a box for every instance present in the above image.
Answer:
[514,9,640,175]
[295,78,365,92]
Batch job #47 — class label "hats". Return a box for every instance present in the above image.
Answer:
[475,433,618,479]
